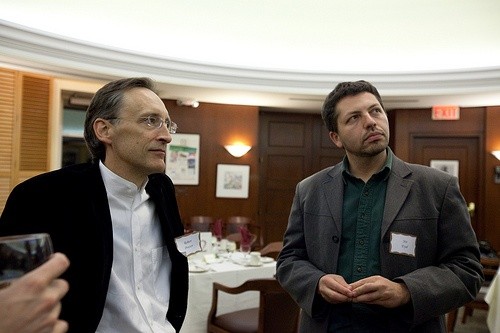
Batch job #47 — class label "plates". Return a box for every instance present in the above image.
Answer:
[245,264,263,266]
[189,269,209,272]
[261,257,273,262]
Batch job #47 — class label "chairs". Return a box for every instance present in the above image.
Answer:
[208,278,300,333]
[222,216,264,252]
[187,215,216,238]
[445,259,500,333]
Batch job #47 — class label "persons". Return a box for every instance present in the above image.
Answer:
[276,80,483,333]
[0,252,71,333]
[0,78,189,333]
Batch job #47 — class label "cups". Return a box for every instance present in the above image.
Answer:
[0,233,53,289]
[250,252,260,264]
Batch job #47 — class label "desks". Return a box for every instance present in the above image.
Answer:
[179,252,277,333]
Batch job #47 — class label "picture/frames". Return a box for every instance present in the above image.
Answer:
[431,160,460,183]
[216,164,250,199]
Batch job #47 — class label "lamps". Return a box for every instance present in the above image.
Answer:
[224,145,252,157]
[491,151,500,160]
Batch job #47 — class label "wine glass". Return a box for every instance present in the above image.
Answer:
[225,242,235,267]
[240,243,250,265]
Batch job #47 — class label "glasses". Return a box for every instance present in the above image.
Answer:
[107,115,177,135]
